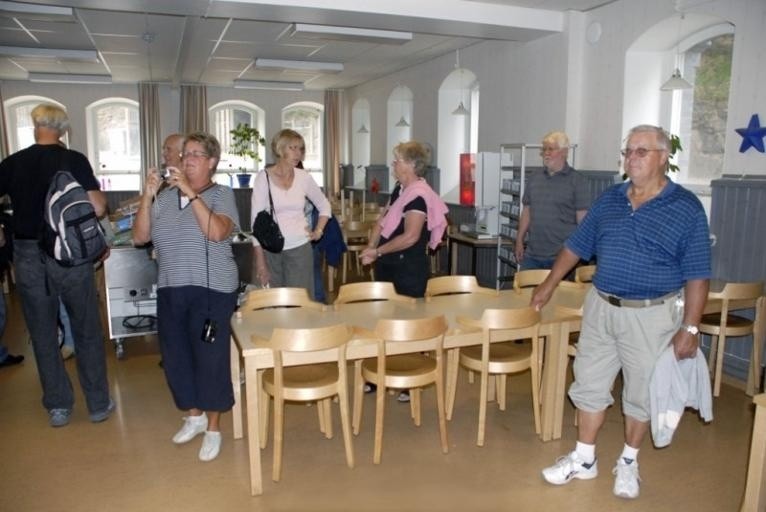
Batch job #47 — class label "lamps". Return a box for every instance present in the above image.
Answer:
[29,73,113,85]
[0,46,97,63]
[0,1,77,23]
[234,81,304,91]
[660,15,693,91]
[451,69,470,116]
[395,88,410,127]
[291,24,413,46]
[357,101,368,133]
[256,59,344,73]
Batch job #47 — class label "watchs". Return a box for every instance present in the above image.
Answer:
[681,324,699,335]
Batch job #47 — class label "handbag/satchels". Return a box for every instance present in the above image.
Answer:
[253,210,284,253]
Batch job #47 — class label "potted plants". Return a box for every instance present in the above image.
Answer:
[228,123,266,187]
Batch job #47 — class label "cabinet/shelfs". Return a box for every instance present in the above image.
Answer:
[497,143,576,290]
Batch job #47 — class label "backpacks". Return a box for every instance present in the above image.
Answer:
[44,171,107,267]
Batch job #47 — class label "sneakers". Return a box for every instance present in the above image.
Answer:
[49,408,72,426]
[542,450,598,485]
[611,457,641,499]
[60,345,75,361]
[199,431,222,461]
[89,397,115,422]
[172,411,209,444]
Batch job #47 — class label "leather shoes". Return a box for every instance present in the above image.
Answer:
[0,354,24,367]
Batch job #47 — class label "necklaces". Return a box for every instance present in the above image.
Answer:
[271,170,292,181]
[184,181,211,196]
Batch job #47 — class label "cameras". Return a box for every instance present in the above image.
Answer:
[152,168,172,181]
[201,318,219,345]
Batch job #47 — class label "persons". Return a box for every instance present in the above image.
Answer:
[358,142,433,403]
[250,130,333,302]
[530,125,713,499]
[514,131,594,344]
[132,133,246,462]
[148,129,185,369]
[1,104,115,428]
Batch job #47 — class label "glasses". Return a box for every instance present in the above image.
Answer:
[178,150,210,159]
[620,148,665,158]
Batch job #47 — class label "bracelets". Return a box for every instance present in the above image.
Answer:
[376,250,382,257]
[190,193,200,202]
[316,227,324,234]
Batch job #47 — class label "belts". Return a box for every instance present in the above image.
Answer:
[598,290,680,308]
